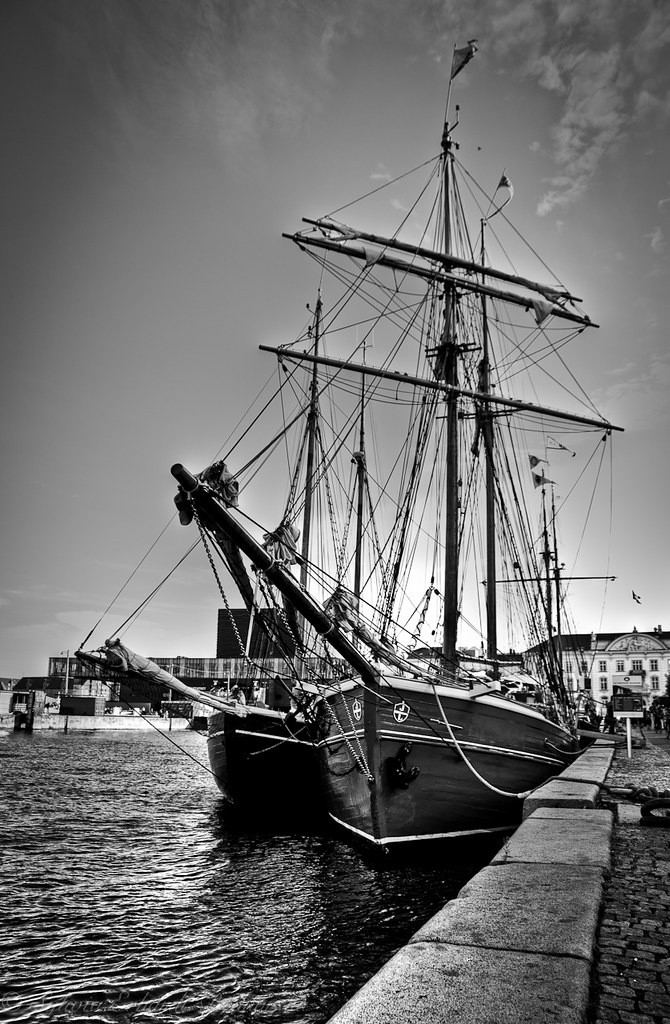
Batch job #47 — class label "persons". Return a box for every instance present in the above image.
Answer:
[648,697,668,734]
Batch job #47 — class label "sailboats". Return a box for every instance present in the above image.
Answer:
[72,287,338,828]
[167,38,627,871]
